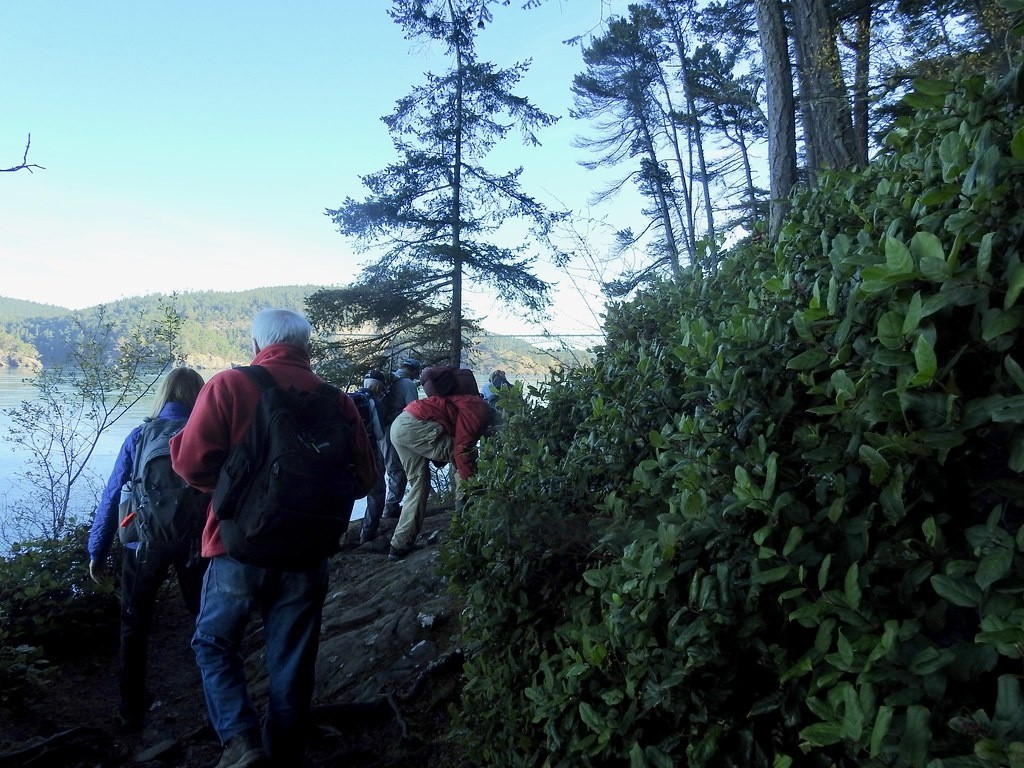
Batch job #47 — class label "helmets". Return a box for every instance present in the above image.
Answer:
[403,359,420,380]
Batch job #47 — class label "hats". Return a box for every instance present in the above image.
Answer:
[364,370,387,394]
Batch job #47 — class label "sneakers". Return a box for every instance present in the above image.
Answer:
[386,506,402,519]
[212,739,259,768]
[387,545,425,562]
[360,531,379,543]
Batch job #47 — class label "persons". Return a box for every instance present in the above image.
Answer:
[388,395,514,560]
[481,370,516,410]
[169,310,377,768]
[359,359,422,544]
[87,366,207,735]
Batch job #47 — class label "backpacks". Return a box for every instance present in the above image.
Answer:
[129,426,143,445]
[345,388,376,448]
[213,365,361,576]
[422,366,481,397]
[117,425,220,564]
